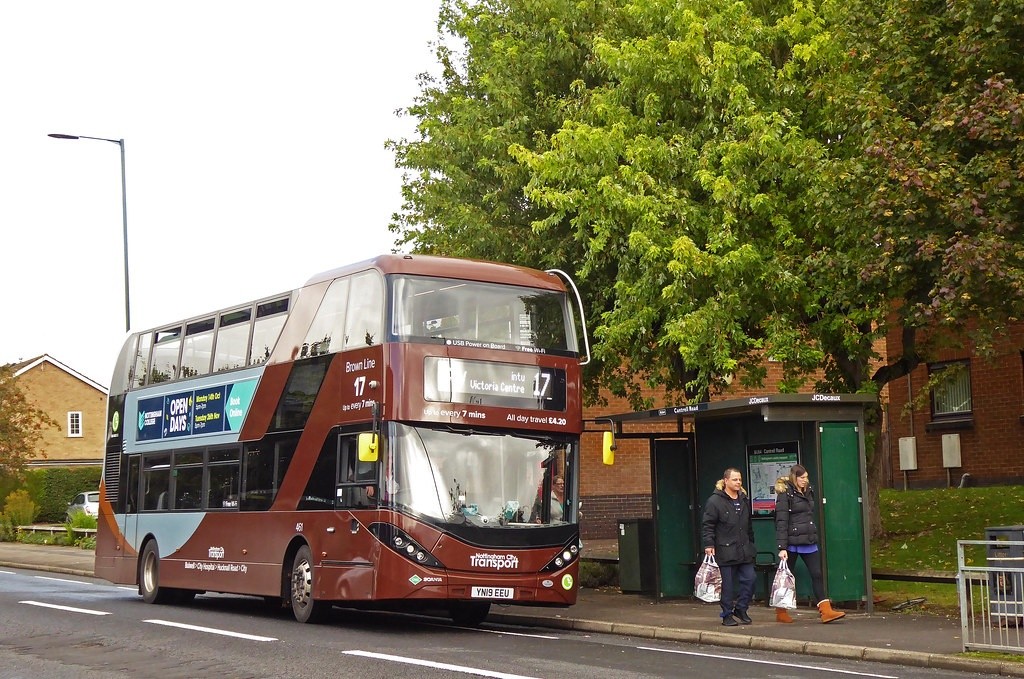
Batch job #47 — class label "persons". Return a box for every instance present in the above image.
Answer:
[703,468,759,627]
[773,464,846,624]
[549,475,584,551]
[350,469,380,496]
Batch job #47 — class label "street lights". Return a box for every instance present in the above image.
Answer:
[48,132,132,333]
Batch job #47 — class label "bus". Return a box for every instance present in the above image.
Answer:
[95,252,618,628]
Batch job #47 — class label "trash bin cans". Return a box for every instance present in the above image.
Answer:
[616,518,656,593]
[983,525,1024,627]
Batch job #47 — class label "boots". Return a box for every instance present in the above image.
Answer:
[775,607,793,622]
[817,598,845,623]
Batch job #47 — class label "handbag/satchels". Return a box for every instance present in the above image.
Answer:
[769,558,797,610]
[693,553,722,603]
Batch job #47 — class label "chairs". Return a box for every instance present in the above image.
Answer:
[156,492,168,509]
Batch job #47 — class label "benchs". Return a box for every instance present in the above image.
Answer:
[679,551,779,607]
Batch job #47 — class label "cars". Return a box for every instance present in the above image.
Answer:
[65,491,99,524]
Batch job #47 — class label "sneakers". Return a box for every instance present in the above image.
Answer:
[722,615,738,626]
[734,610,752,623]
